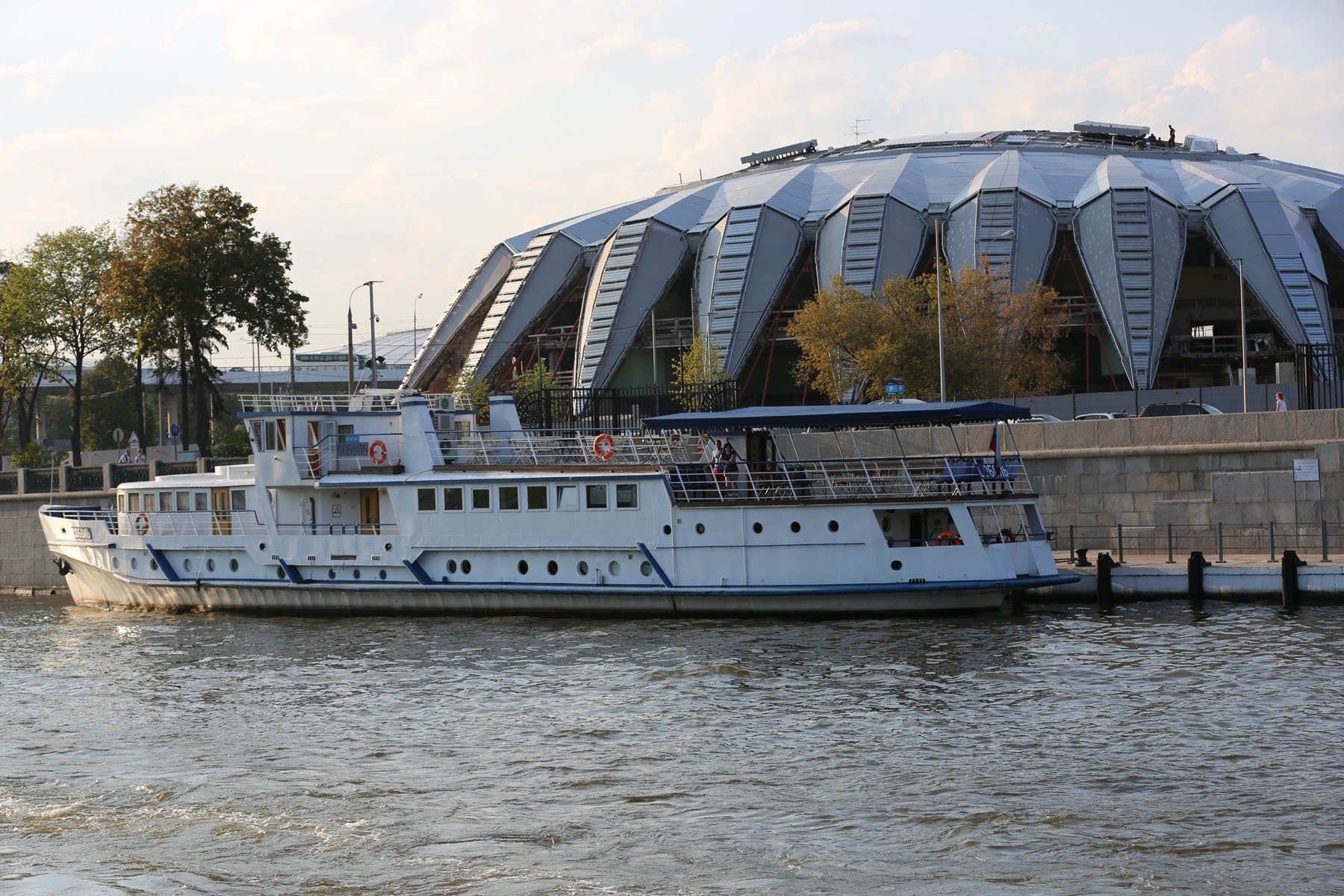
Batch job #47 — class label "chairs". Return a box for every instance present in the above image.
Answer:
[749,457,1020,500]
[462,433,692,465]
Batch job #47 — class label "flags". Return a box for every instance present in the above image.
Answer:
[990,423,1002,477]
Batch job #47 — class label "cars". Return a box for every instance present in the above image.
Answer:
[1071,411,1129,420]
[996,413,1065,423]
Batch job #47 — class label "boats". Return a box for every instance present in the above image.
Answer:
[38,281,1085,617]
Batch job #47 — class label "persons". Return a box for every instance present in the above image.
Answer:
[1275,392,1287,412]
[698,433,742,504]
[119,448,146,482]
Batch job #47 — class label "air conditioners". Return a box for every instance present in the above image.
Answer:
[435,397,454,410]
[436,433,458,460]
[434,414,455,431]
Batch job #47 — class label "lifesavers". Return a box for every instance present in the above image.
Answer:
[440,438,451,454]
[996,528,1015,543]
[135,514,149,535]
[593,433,614,460]
[369,440,387,465]
[935,531,961,545]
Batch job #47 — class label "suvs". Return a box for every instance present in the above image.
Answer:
[229,367,250,372]
[1139,400,1225,418]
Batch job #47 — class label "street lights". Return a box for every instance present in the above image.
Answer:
[639,306,658,386]
[348,280,385,395]
[916,216,957,403]
[414,293,424,358]
[983,229,1016,285]
[690,255,718,412]
[1228,257,1249,413]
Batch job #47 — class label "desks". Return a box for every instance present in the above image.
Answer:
[551,438,578,462]
[513,439,552,465]
[464,440,502,464]
[639,437,670,464]
[587,438,626,463]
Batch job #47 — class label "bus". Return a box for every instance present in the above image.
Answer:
[292,353,372,371]
